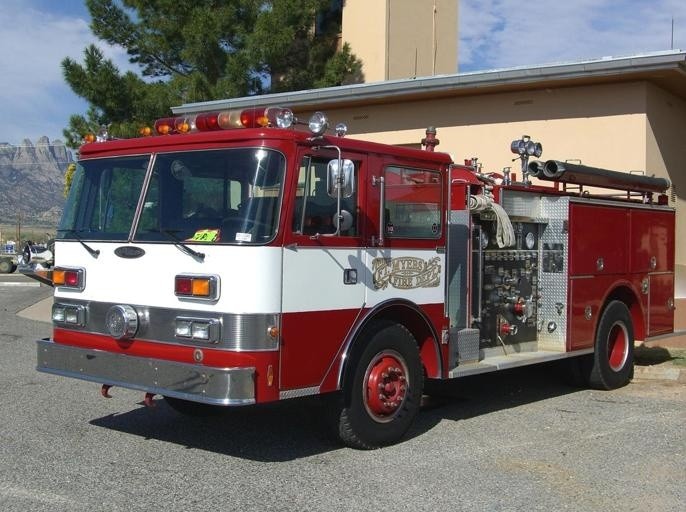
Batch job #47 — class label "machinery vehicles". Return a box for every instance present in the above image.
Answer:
[0,238,52,286]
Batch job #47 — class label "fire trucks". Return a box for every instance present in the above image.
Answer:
[18,106,685,452]
